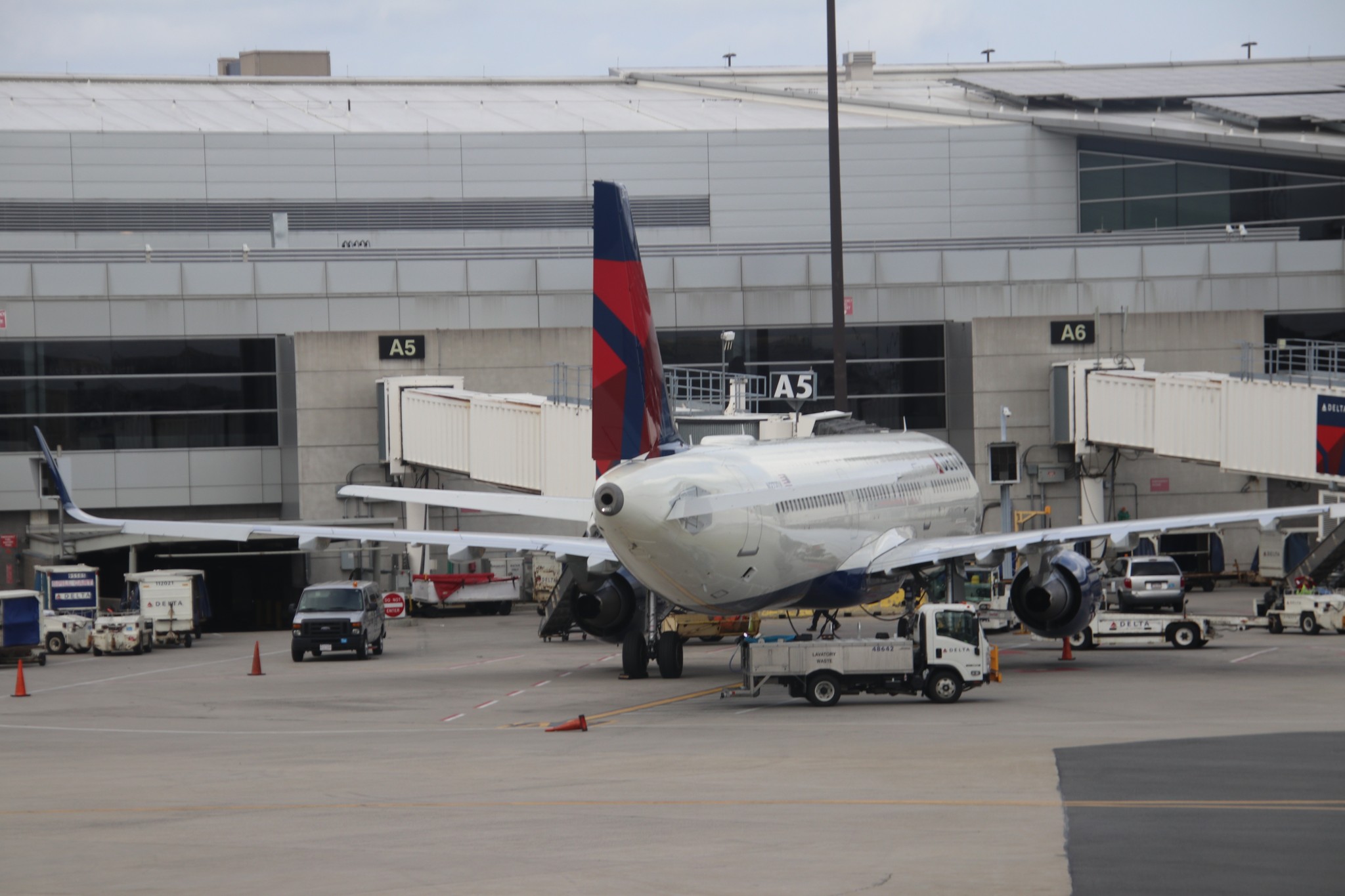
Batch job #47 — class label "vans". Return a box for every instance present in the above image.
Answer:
[1100,555,1186,613]
[291,579,385,663]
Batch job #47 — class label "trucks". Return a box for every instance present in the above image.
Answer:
[740,602,994,707]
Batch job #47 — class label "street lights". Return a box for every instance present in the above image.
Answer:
[718,330,735,416]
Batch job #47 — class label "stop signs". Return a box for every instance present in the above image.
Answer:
[381,593,405,617]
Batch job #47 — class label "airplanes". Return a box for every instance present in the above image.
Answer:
[39,177,1345,626]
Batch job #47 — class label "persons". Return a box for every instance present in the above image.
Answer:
[806,608,841,631]
[1294,575,1316,595]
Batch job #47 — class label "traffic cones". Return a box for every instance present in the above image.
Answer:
[1059,636,1077,661]
[9,659,33,698]
[246,641,267,676]
[544,714,588,733]
